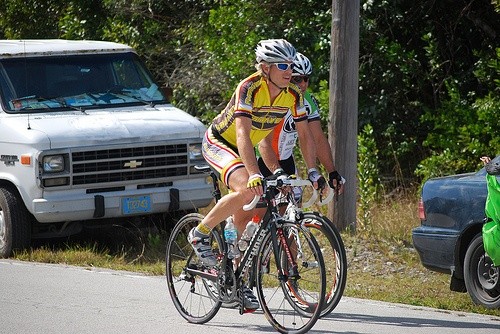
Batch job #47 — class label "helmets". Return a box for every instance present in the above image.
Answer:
[291,50,313,76]
[255,38,297,63]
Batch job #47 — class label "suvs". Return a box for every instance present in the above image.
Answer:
[0,39,217,259]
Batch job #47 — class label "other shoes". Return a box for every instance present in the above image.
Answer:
[282,268,301,298]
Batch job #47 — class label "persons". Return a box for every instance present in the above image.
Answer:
[186,38,328,310]
[251,52,343,295]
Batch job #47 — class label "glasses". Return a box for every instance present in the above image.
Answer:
[273,62,294,71]
[293,76,311,83]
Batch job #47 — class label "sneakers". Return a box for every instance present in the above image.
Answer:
[236,283,260,308]
[186,226,217,266]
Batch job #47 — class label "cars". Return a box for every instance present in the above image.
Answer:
[410,157,500,310]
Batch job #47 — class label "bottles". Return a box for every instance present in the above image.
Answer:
[225,218,240,260]
[238,215,262,252]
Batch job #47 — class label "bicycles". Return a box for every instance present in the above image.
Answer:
[201,179,348,317]
[165,180,326,334]
